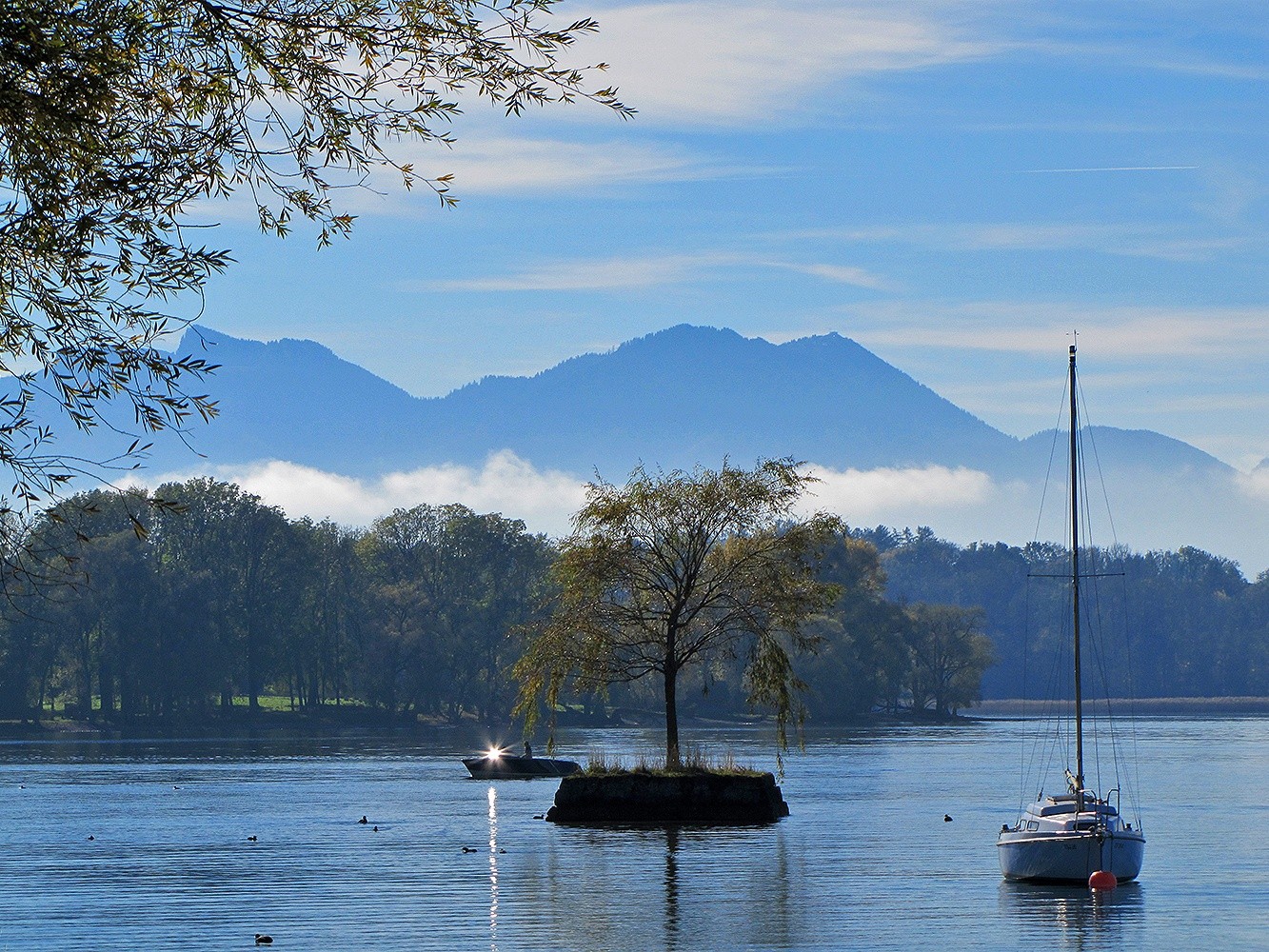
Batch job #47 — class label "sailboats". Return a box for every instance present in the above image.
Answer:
[997,329,1147,884]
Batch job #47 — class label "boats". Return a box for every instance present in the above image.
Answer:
[461,751,580,777]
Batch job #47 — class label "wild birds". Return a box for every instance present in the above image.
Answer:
[247,836,257,841]
[255,933,273,944]
[533,814,545,819]
[174,786,180,790]
[21,785,26,788]
[944,814,952,822]
[357,815,367,824]
[462,846,477,853]
[498,848,506,853]
[373,826,378,831]
[88,835,94,840]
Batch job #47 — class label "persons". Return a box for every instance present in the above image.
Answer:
[520,741,532,759]
